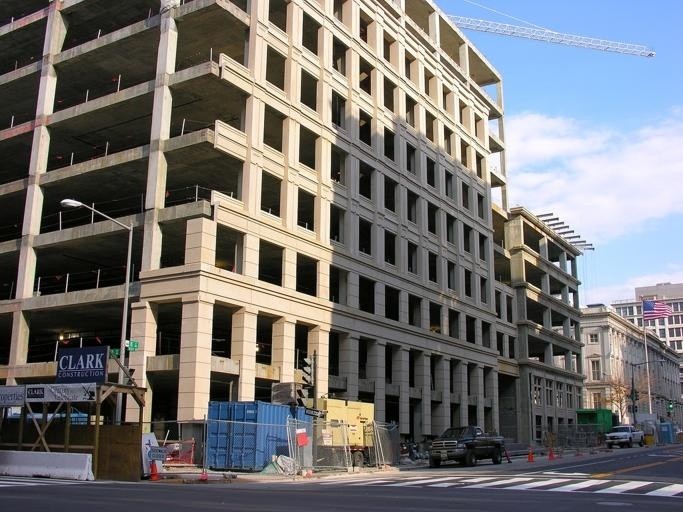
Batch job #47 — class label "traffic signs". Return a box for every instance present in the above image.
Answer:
[124,339,139,348]
[110,348,120,358]
[127,347,137,352]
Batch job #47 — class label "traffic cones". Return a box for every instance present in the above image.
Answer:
[526,446,536,462]
[150,462,160,480]
[549,447,555,461]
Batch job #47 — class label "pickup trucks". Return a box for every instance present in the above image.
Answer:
[606,425,646,449]
[428,425,505,467]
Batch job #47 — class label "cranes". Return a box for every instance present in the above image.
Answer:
[446,0,656,58]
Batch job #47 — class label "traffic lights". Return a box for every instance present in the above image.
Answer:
[301,357,314,385]
[296,387,308,408]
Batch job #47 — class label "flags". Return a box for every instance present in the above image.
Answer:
[643,300,673,321]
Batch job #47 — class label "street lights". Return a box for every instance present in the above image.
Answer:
[631,359,667,426]
[60,197,137,425]
